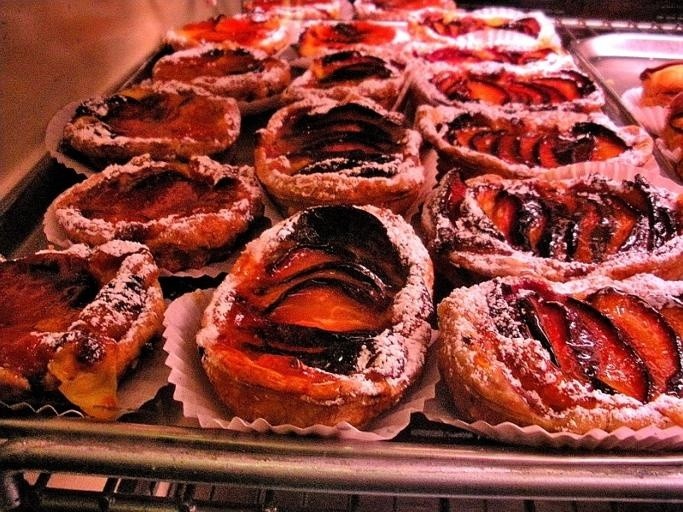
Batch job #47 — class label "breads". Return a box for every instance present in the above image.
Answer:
[0,1,683,430]
